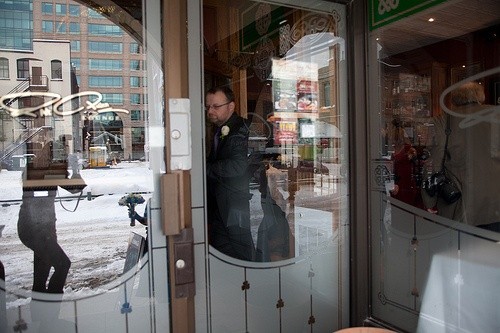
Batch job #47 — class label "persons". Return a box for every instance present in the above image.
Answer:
[387,122,427,207]
[208,84,261,264]
[17,140,84,293]
[421,83,500,233]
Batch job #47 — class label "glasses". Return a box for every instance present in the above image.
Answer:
[204,101,232,110]
